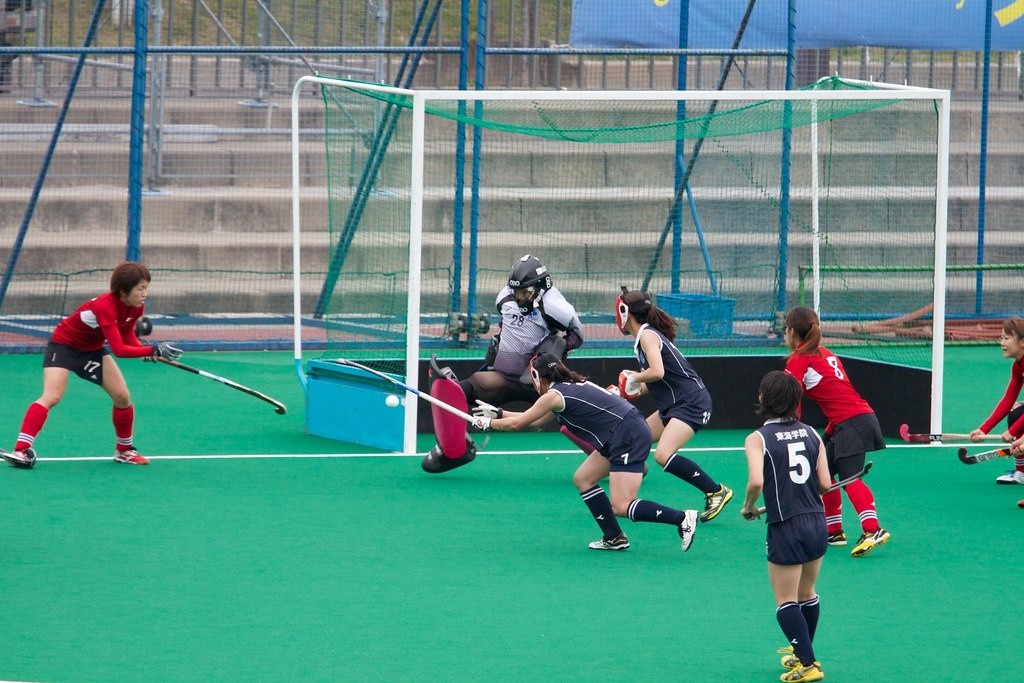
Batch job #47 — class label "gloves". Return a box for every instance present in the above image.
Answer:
[472,415,494,432]
[157,342,182,361]
[472,399,503,419]
[141,356,157,363]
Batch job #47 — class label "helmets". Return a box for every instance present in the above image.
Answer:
[506,254,552,316]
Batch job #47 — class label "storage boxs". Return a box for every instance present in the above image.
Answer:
[657,292,737,341]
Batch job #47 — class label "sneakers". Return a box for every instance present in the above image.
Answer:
[13,452,30,464]
[114,447,149,465]
[996,470,1024,484]
[780,662,824,683]
[851,528,891,556]
[678,510,699,551]
[588,532,630,550]
[776,646,823,672]
[827,531,847,545]
[700,484,734,522]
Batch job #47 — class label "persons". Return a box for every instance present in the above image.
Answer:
[969,315,1024,507]
[422,254,596,473]
[471,353,700,551]
[784,307,891,557]
[11,261,184,465]
[740,371,832,683]
[605,286,734,523]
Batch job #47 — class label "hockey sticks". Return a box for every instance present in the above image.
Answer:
[743,460,873,519]
[465,431,490,451]
[335,357,474,423]
[154,356,287,415]
[958,444,1024,465]
[900,424,1017,441]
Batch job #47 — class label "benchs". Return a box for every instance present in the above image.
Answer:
[0,86,1024,318]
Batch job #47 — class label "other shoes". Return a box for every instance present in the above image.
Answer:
[1018,500,1024,507]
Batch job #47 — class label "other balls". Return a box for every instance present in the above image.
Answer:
[385,394,400,408]
